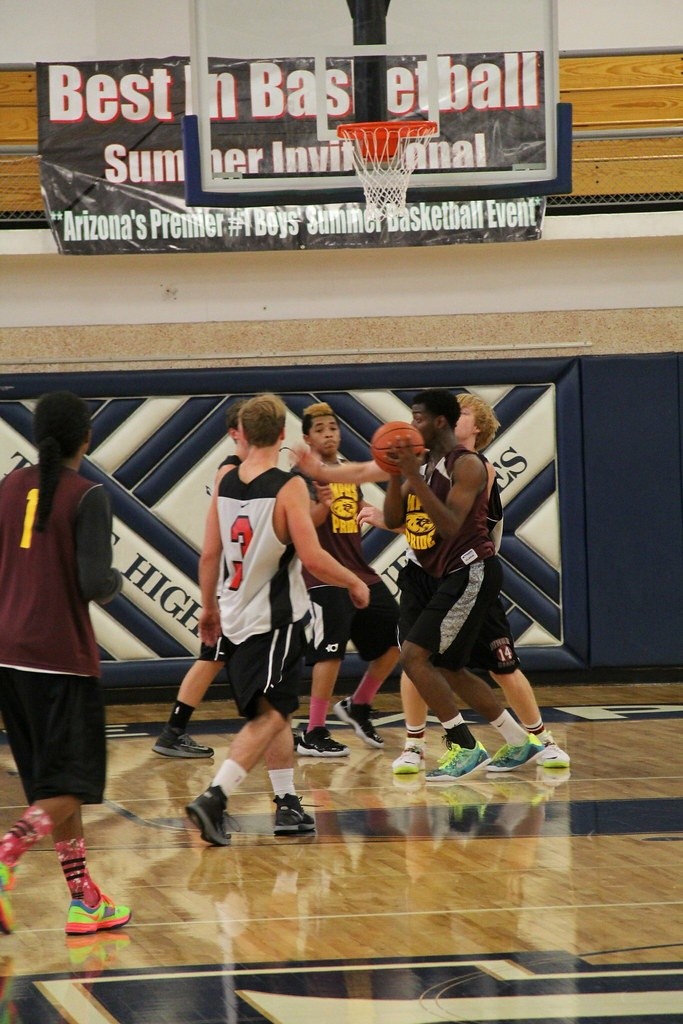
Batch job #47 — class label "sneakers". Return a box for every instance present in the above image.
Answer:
[341,751,384,802]
[0,953,14,1024]
[154,754,215,781]
[333,696,384,749]
[152,727,215,758]
[186,786,240,845]
[428,738,492,784]
[297,756,350,786]
[293,733,302,750]
[487,774,554,807]
[534,734,570,769]
[296,729,351,758]
[537,764,570,789]
[66,882,132,933]
[392,744,426,774]
[65,935,131,964]
[485,733,543,771]
[0,859,15,935]
[272,793,316,835]
[424,779,494,811]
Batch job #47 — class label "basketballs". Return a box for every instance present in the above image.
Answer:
[372,421,426,473]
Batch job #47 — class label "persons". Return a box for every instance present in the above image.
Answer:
[385,387,545,784]
[292,400,404,757]
[391,392,575,776]
[0,391,132,935]
[183,392,372,846]
[151,390,252,761]
